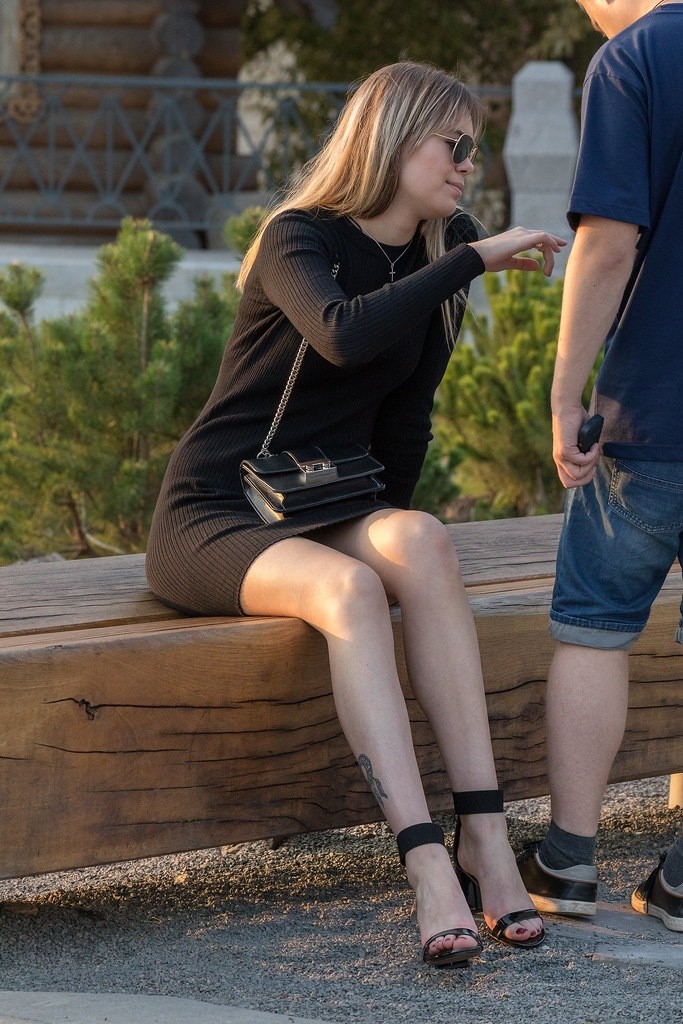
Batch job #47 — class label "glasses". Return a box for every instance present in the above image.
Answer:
[432,132,478,165]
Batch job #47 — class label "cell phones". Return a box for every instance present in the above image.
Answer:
[578,413,605,455]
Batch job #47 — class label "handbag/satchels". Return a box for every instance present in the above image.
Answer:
[238,439,385,525]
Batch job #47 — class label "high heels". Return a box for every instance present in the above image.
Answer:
[396,822,484,965]
[452,789,546,947]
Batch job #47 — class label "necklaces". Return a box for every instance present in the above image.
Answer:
[354,219,413,282]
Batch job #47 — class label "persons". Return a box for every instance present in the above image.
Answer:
[516,0,683,932]
[144,63,546,965]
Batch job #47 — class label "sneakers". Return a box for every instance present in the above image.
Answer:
[631,851,683,931]
[513,839,599,916]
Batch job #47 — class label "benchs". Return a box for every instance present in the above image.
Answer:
[0,514,683,876]
